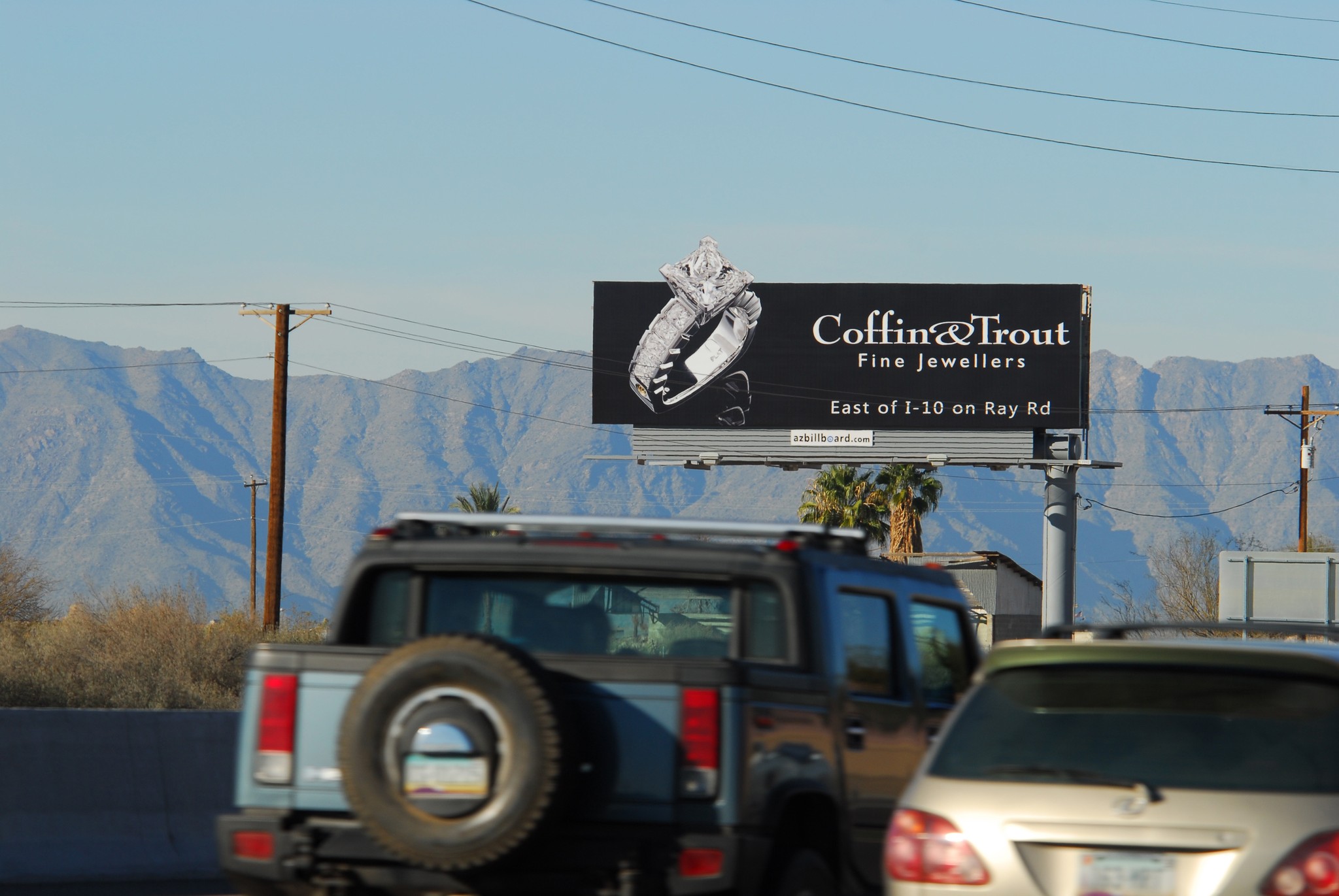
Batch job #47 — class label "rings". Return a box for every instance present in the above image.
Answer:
[628,236,763,413]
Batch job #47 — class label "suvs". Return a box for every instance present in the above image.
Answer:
[210,506,985,896]
[872,613,1338,896]
